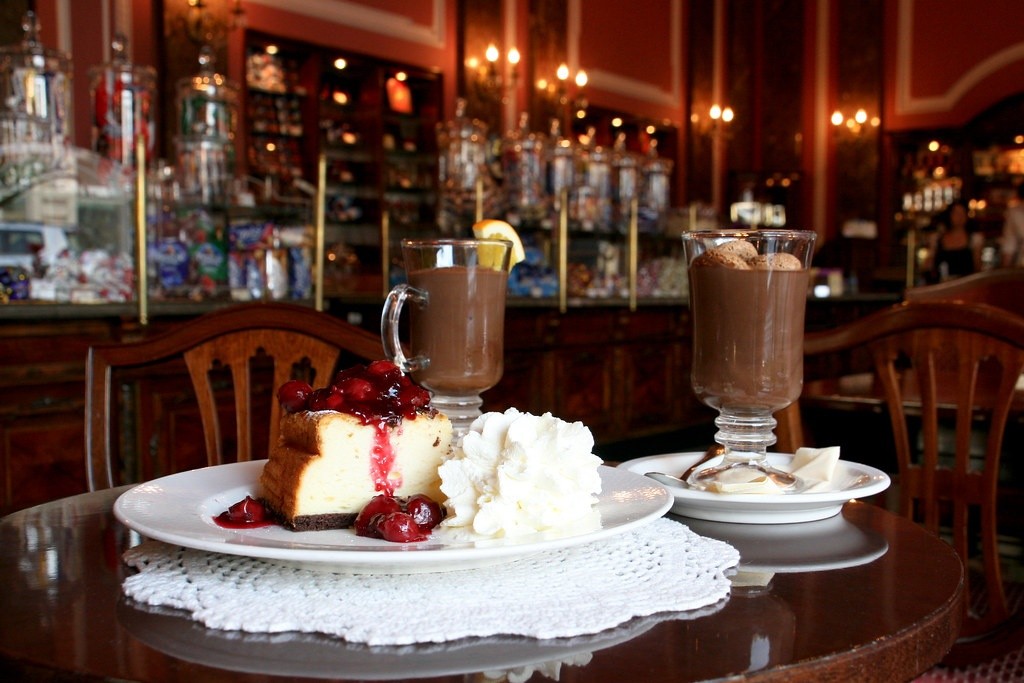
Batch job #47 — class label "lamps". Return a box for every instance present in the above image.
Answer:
[541,62,589,113]
[831,108,880,168]
[475,43,521,100]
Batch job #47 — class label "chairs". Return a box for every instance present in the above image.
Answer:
[773,301,1024,683]
[83,302,410,495]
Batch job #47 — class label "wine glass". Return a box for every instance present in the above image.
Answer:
[381,236,515,457]
[680,228,818,494]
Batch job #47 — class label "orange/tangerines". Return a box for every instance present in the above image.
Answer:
[473,218,525,273]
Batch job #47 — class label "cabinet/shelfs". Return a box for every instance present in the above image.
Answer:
[227,29,444,225]
[878,123,1024,283]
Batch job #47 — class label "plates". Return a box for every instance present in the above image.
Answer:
[616,453,892,524]
[111,458,675,570]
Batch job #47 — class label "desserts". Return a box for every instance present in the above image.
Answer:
[218,359,603,545]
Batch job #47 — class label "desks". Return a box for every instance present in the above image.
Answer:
[0,485,965,683]
